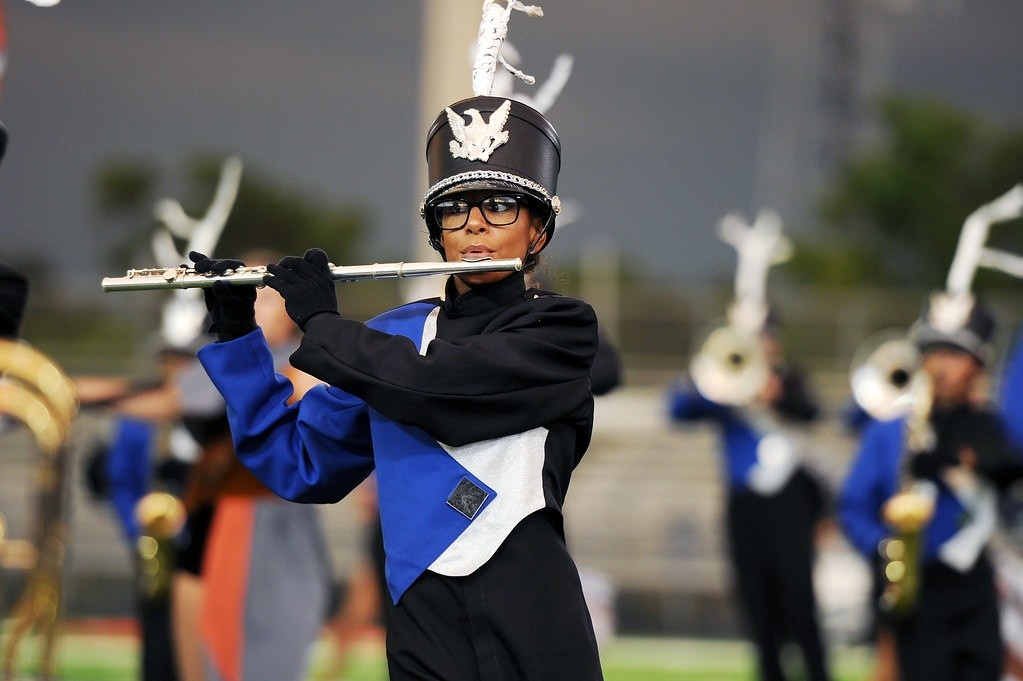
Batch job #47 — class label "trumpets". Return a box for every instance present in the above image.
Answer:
[846,326,927,424]
[690,324,773,408]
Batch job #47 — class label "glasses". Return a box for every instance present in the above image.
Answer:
[433,194,533,230]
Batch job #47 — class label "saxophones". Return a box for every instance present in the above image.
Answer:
[884,373,941,614]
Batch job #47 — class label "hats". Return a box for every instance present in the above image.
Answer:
[915,310,992,366]
[419,0,574,258]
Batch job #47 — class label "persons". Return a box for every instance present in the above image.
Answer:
[188,98,615,681]
[662,299,832,681]
[0,241,328,681]
[837,285,1023,680]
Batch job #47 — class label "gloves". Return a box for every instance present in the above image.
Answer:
[181,252,258,341]
[263,248,340,332]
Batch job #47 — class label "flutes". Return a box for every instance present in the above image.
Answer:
[100,256,525,297]
